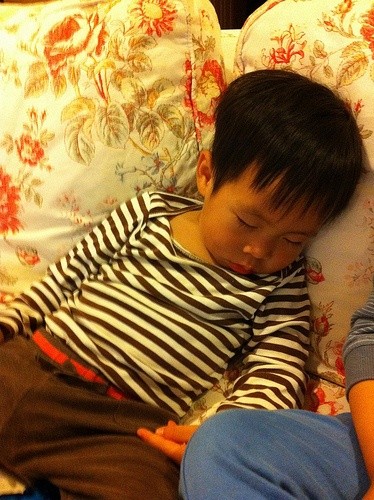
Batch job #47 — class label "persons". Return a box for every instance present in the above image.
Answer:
[179,277,374,500]
[0,69,362,500]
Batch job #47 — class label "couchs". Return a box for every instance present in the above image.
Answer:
[0,0,374,426]
[0,0,374,500]
